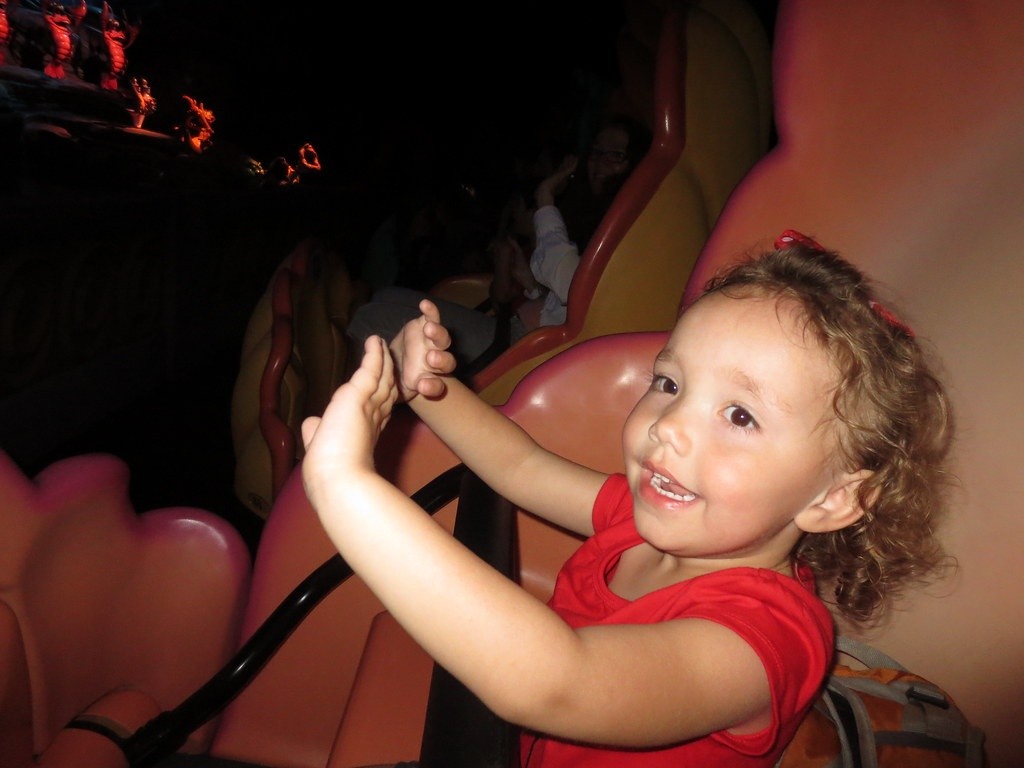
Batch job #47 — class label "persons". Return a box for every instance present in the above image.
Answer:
[302,235,956,768]
[342,117,649,389]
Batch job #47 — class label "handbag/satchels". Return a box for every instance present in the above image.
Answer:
[771,636,988,768]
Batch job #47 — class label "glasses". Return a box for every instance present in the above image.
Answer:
[586,148,630,164]
